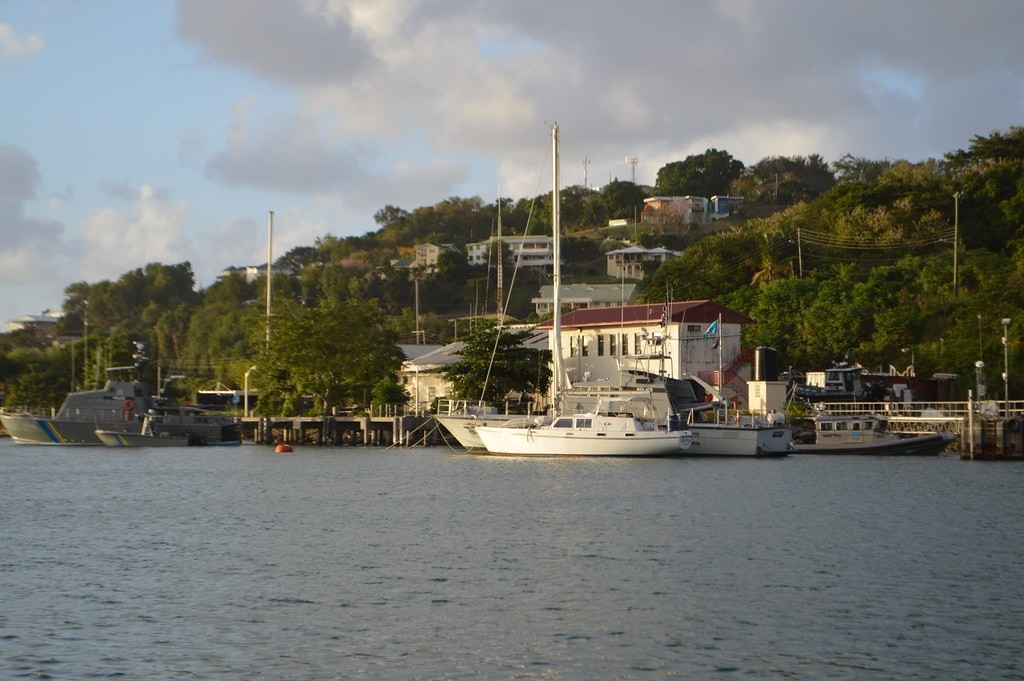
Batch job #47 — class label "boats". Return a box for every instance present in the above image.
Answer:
[475,414,694,458]
[95,414,192,446]
[0,341,243,447]
[796,368,887,402]
[792,416,957,455]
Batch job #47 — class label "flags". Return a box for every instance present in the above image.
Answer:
[704,320,717,349]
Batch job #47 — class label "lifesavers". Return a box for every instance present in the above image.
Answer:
[124,400,133,411]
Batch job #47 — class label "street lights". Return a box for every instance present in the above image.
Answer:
[414,279,419,345]
[1002,318,1011,416]
[953,192,961,299]
[448,319,457,339]
[265,211,274,348]
[901,348,914,365]
[244,365,257,417]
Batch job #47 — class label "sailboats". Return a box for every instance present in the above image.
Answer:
[432,121,794,457]
